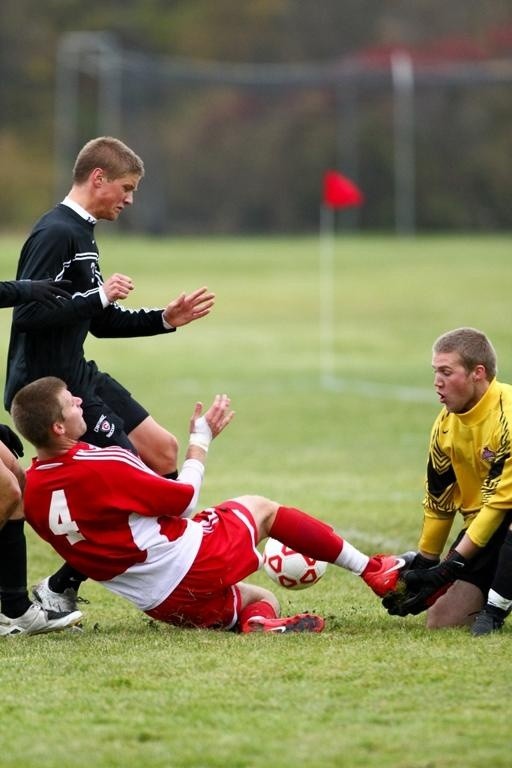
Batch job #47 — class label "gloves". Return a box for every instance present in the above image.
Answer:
[32,278,72,311]
[382,551,467,616]
[0,424,24,459]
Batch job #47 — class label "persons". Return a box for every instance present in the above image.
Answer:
[383,328,511,633]
[9,375,417,633]
[1,278,85,637]
[3,138,215,626]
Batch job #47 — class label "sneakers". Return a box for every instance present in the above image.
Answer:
[243,614,325,634]
[364,551,417,598]
[0,601,84,637]
[468,610,505,637]
[32,575,82,628]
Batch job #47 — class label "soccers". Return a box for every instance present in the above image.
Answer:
[262,537,328,590]
